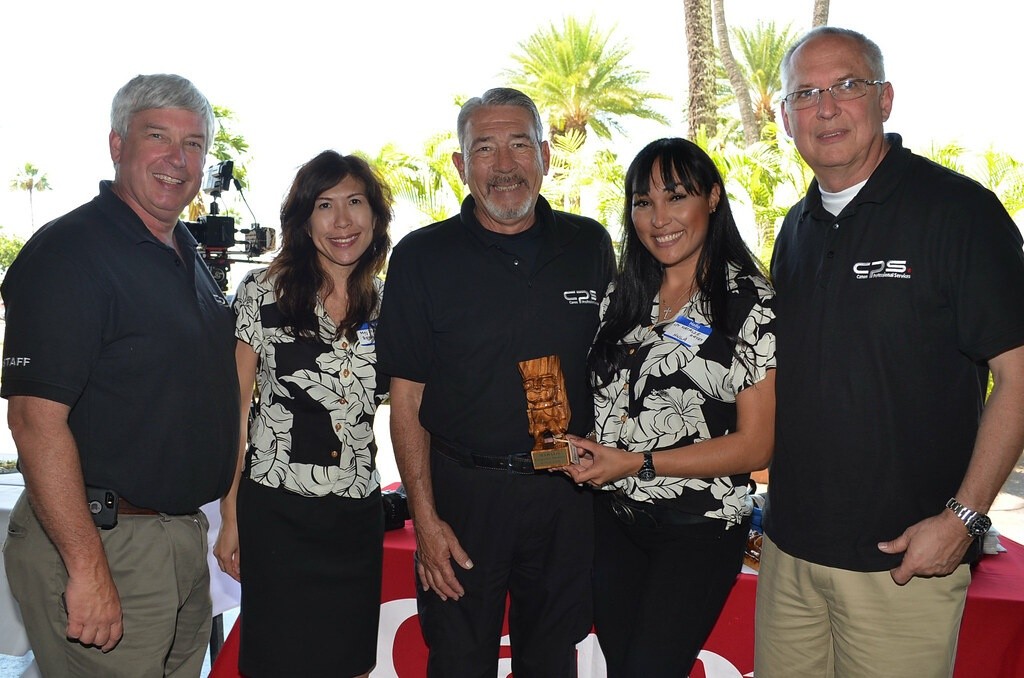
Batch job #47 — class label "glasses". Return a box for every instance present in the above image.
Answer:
[781,79,884,111]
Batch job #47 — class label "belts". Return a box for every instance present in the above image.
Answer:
[115,497,200,515]
[429,435,540,477]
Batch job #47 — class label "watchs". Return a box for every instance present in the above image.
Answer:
[637,451,656,481]
[946,497,992,538]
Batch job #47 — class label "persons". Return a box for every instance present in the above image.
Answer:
[753,27,1024,678]
[212,149,391,678]
[550,137,775,678]
[0,74,242,678]
[376,88,618,678]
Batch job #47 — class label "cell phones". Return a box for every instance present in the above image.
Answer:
[85,488,117,527]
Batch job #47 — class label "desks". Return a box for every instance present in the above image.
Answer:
[204,478,1022,676]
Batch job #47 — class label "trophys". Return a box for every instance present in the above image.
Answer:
[517,356,578,470]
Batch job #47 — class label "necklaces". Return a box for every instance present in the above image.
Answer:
[663,288,691,320]
